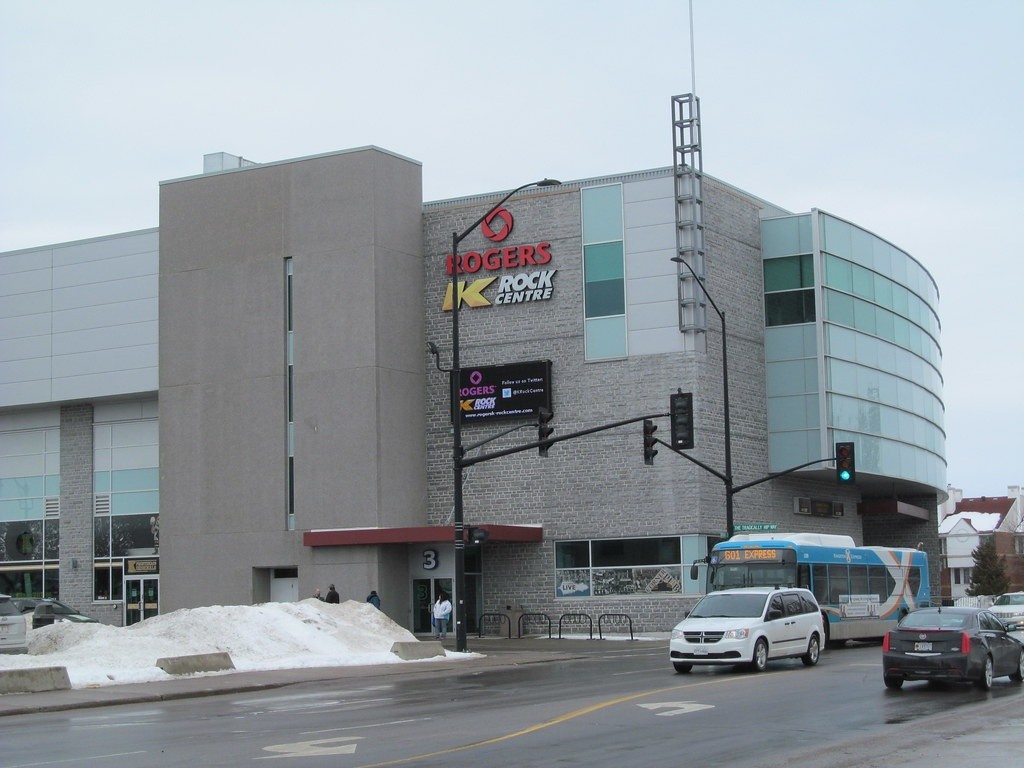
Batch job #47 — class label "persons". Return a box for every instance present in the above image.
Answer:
[367,591,380,610]
[432,594,452,640]
[313,584,340,604]
[634,574,650,590]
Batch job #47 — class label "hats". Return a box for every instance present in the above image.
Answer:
[371,591,378,595]
[328,584,335,588]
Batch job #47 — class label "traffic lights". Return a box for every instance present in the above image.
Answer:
[835,441,856,485]
[536,406,554,457]
[643,419,659,467]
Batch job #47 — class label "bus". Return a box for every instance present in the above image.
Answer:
[689,532,932,649]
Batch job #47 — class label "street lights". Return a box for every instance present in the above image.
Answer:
[453,178,562,652]
[667,255,736,539]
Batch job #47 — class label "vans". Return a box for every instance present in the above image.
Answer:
[669,585,826,674]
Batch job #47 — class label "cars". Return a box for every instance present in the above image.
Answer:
[986,591,1024,629]
[881,606,1024,691]
[0,594,29,655]
[11,597,99,631]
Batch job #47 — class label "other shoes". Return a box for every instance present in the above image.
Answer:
[434,636,439,640]
[441,636,446,640]
